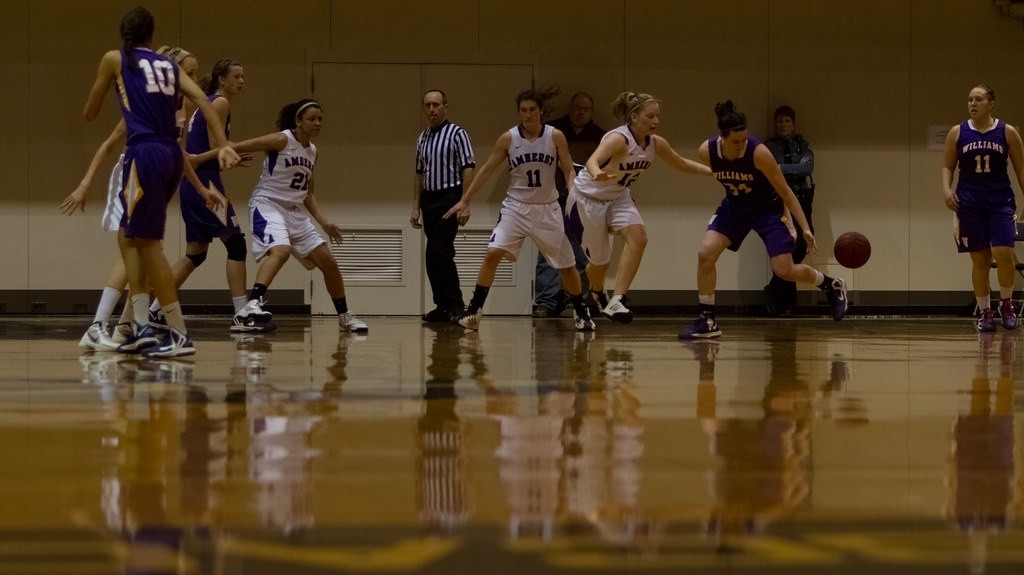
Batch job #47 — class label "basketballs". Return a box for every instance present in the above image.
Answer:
[833,231,872,269]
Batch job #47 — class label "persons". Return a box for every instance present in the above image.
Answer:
[83,6,241,358]
[58,45,225,352]
[535,93,611,319]
[148,58,277,332]
[942,84,1024,331]
[185,99,369,332]
[574,91,713,322]
[442,77,595,330]
[679,98,848,338]
[762,105,814,317]
[409,89,476,323]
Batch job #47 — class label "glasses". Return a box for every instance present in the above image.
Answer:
[570,104,592,113]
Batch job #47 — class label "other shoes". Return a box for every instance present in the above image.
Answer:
[422,303,465,322]
[763,285,777,317]
[784,305,795,317]
[533,305,548,317]
[561,304,574,317]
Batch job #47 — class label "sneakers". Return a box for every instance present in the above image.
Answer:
[339,312,368,332]
[233,299,273,321]
[602,294,633,324]
[230,315,277,331]
[142,327,195,357]
[978,308,996,331]
[118,324,159,353]
[79,321,122,351]
[573,302,596,330]
[823,278,848,321]
[450,304,482,330]
[591,289,614,322]
[998,298,1018,330]
[978,333,995,349]
[678,314,723,338]
[112,322,134,343]
[1000,333,1015,361]
[677,336,720,361]
[149,307,169,329]
